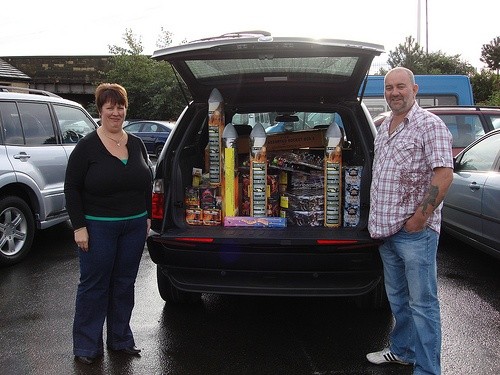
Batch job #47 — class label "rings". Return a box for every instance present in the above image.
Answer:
[78,244,80,247]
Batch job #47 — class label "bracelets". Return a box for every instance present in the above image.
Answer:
[74,227,87,233]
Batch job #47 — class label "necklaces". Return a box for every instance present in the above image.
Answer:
[101,129,125,147]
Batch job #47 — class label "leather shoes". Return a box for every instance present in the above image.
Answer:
[73,355,95,366]
[108,346,141,355]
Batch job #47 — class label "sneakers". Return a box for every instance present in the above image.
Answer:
[365,346,412,366]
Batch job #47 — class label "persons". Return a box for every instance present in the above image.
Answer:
[63,82,153,365]
[367,67,453,375]
